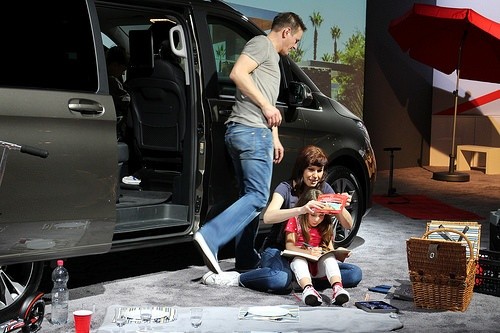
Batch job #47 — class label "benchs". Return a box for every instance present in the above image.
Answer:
[456,143,500,175]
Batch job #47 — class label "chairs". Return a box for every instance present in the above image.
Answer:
[128,20,188,151]
[106,46,130,141]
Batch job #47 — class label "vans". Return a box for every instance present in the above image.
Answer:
[0,0,378,326]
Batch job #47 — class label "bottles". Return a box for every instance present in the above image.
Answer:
[51,260,69,325]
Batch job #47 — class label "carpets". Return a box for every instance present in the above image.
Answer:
[371,193,487,221]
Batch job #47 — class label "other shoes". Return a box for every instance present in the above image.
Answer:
[303,285,323,306]
[203,270,240,288]
[235,257,263,273]
[193,231,221,274]
[331,284,350,306]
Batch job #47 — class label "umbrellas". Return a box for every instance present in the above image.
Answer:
[387,4,500,172]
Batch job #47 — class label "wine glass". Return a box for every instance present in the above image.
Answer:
[140,303,152,331]
[115,307,128,333]
[189,308,203,333]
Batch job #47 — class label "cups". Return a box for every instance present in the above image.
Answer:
[72,310,92,333]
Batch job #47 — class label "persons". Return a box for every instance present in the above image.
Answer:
[192,12,307,272]
[285,189,350,306]
[201,145,362,296]
[106,46,132,112]
